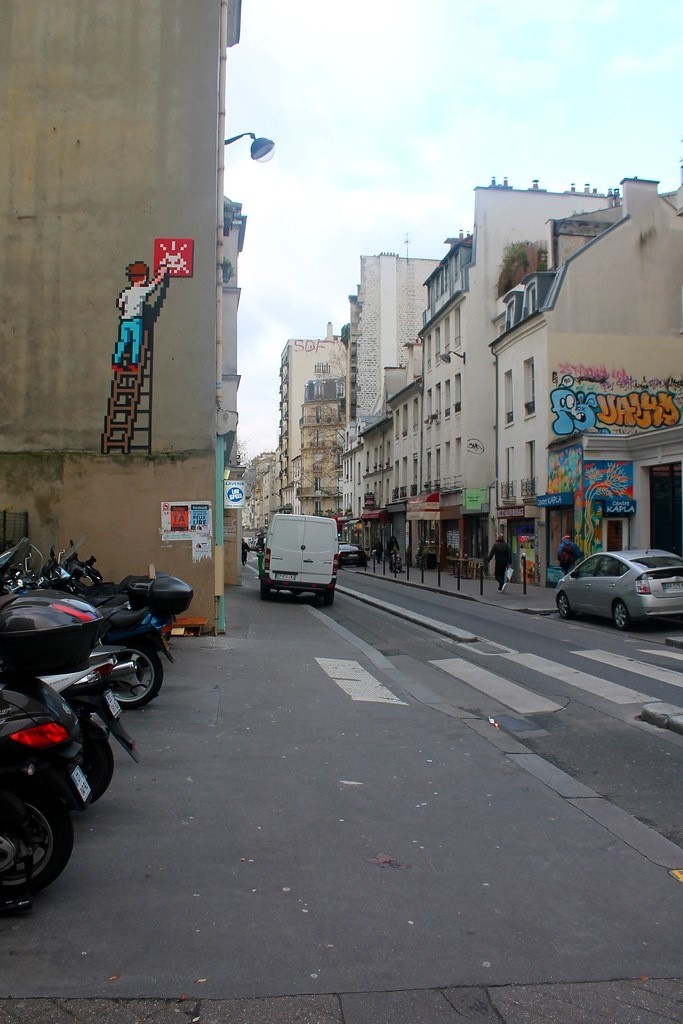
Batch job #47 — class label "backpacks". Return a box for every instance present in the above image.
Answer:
[560,543,573,568]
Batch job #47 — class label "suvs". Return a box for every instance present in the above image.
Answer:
[338,544,367,570]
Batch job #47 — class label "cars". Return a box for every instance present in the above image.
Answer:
[556,548,683,630]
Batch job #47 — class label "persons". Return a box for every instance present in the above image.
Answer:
[484,533,513,593]
[373,538,383,564]
[387,536,400,572]
[557,535,583,576]
[241,538,250,566]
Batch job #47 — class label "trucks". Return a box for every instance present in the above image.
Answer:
[259,514,339,607]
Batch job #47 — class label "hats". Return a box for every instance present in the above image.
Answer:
[562,535,571,540]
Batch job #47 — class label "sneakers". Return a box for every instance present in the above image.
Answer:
[501,583,507,592]
[497,588,501,592]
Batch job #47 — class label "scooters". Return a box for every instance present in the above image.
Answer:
[0,642,142,810]
[1,588,106,898]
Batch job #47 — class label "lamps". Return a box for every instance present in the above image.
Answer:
[438,349,466,366]
[330,442,343,455]
[224,133,276,163]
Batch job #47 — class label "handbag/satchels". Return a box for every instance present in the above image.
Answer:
[505,567,514,580]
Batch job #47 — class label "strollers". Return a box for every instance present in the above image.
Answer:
[391,551,405,573]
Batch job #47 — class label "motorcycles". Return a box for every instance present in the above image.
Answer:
[0,534,193,711]
